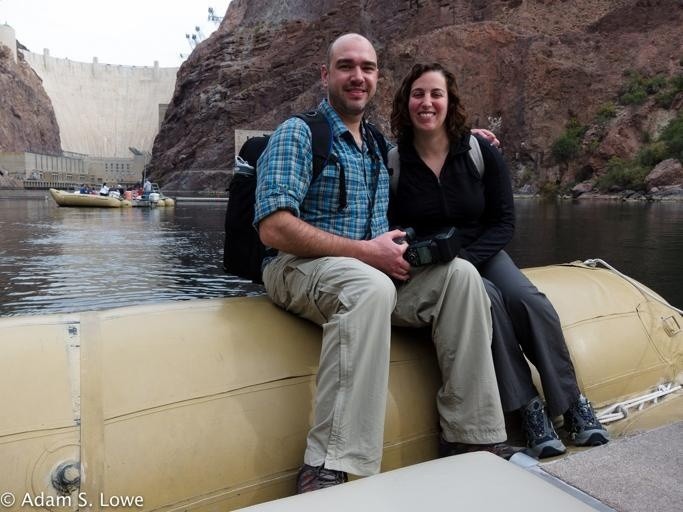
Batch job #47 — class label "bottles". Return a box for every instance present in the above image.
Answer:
[234,156,253,176]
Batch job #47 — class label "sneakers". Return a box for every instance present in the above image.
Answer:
[296,464,347,494]
[438,434,514,459]
[564,396,609,447]
[522,396,567,458]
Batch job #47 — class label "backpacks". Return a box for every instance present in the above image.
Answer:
[223,111,388,284]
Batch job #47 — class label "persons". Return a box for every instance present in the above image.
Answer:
[253,33,514,495]
[79,178,152,196]
[386,62,609,459]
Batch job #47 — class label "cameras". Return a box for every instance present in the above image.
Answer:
[392,225,464,268]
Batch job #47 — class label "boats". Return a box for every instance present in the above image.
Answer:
[0,260,683,512]
[49,187,175,208]
